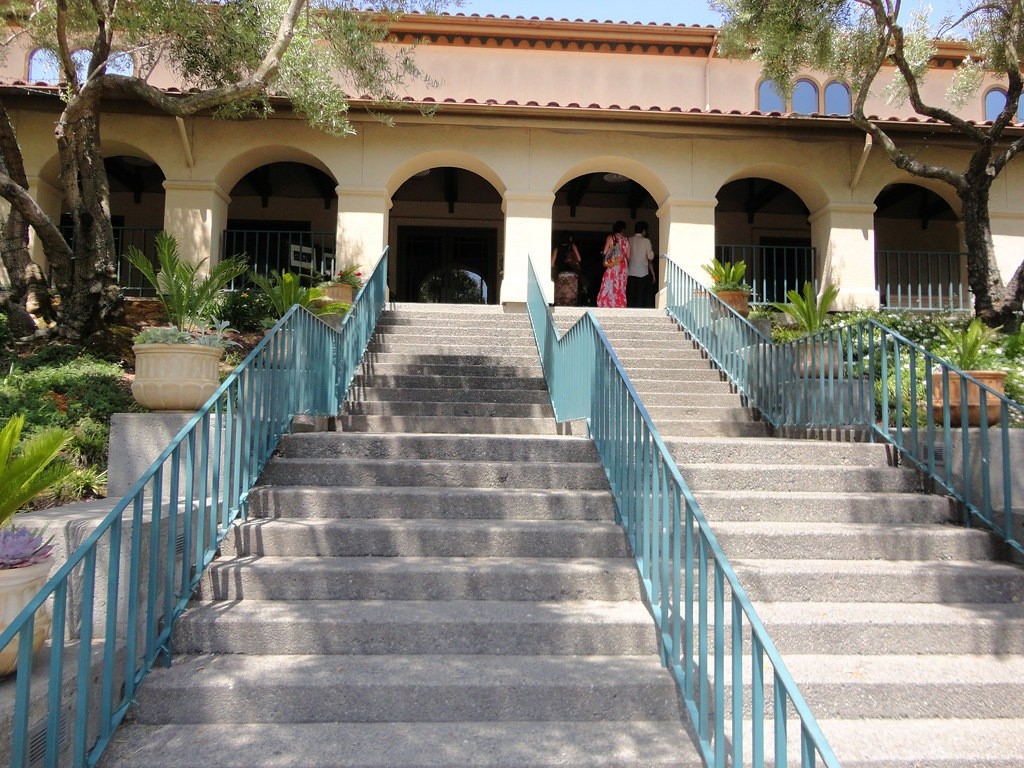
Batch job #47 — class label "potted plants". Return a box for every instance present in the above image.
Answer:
[0,413,76,681]
[122,229,248,410]
[773,281,840,377]
[248,268,357,368]
[701,257,749,319]
[931,317,1007,426]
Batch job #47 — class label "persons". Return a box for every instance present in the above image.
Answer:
[551,229,582,306]
[596,218,656,308]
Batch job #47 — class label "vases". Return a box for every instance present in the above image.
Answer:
[316,283,352,307]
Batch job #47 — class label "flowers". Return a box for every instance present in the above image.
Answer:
[0,523,56,568]
[299,262,363,289]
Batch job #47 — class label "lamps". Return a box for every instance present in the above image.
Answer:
[603,173,629,182]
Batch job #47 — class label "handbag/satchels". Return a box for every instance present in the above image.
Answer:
[603,234,623,267]
[567,243,578,267]
